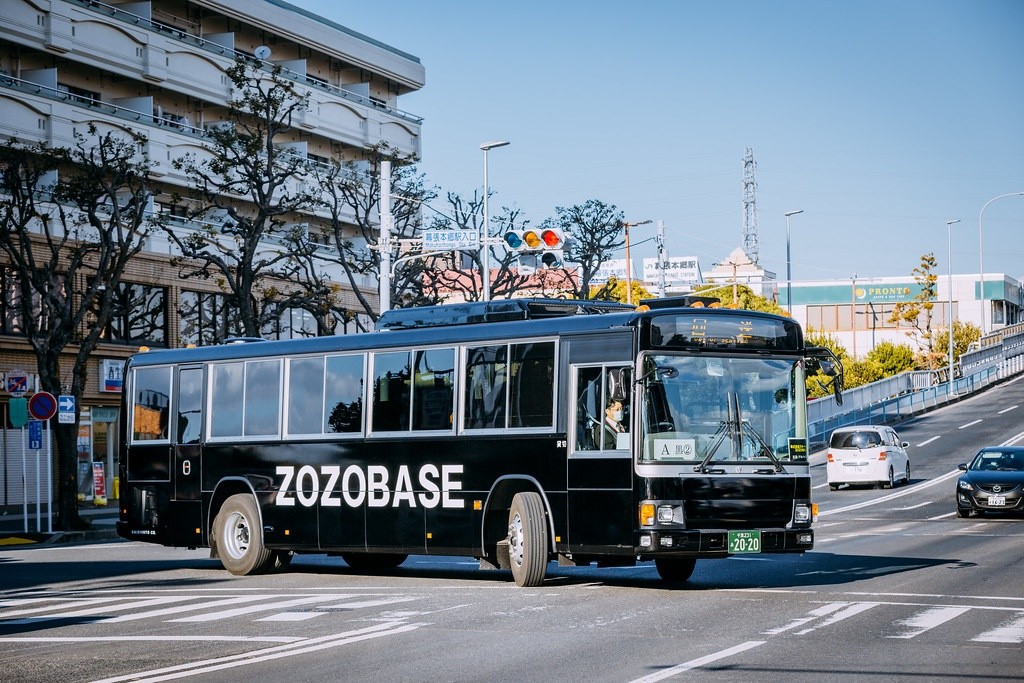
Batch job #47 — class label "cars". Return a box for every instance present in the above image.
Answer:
[826,424,910,491]
[956,445,1024,518]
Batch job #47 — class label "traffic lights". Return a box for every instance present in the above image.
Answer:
[542,250,565,271]
[504,229,566,252]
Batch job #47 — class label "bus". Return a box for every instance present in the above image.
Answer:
[112,295,844,587]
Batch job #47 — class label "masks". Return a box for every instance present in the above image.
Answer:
[609,411,623,422]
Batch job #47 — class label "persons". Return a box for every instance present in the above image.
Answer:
[595,397,626,450]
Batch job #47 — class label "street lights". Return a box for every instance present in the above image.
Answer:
[947,219,961,395]
[624,219,653,304]
[979,192,1024,337]
[785,209,805,314]
[480,139,512,301]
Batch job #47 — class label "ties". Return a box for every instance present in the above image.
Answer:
[617,425,622,433]
